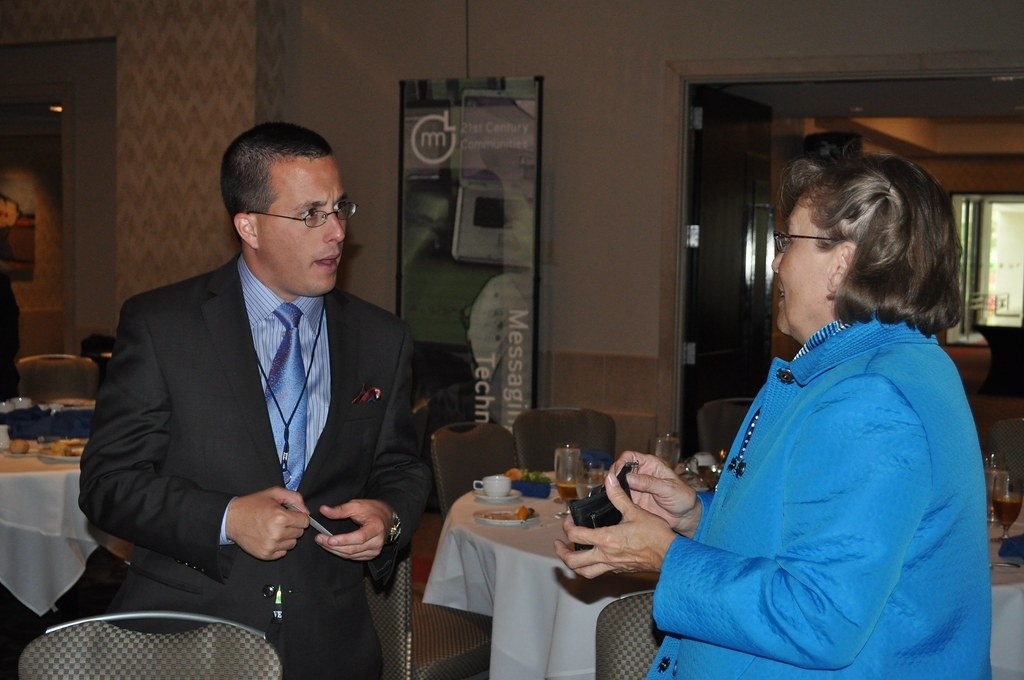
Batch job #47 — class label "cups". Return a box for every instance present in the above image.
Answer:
[574,460,604,500]
[473,476,512,497]
[0,397,32,414]
[648,432,680,472]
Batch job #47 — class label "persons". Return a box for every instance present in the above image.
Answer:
[556,154,993,679]
[77,120,435,680]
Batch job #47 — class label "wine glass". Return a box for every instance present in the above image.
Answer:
[990,474,1023,543]
[983,450,1009,523]
[552,441,580,522]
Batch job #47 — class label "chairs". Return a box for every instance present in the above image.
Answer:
[513,407,614,471]
[19,611,284,680]
[431,422,517,520]
[365,548,492,680]
[16,353,100,401]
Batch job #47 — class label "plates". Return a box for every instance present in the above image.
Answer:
[2,448,37,458]
[38,453,81,463]
[475,489,522,502]
[473,509,540,525]
[691,487,709,492]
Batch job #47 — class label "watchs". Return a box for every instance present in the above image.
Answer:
[389,516,401,542]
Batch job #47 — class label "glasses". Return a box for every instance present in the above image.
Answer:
[773,230,838,252]
[246,201,359,228]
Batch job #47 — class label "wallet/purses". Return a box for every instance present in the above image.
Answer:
[569,460,639,550]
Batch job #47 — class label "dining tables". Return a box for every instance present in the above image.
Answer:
[423,461,1023,680]
[696,398,754,452]
[0,403,131,614]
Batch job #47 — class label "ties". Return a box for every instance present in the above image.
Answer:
[265,302,308,492]
